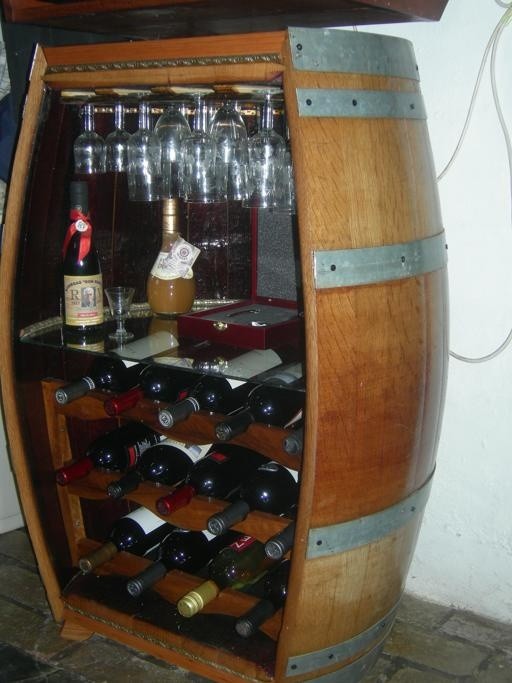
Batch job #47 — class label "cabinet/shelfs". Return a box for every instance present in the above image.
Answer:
[23,298,305,664]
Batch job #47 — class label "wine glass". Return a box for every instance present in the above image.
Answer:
[71,94,294,217]
[106,286,134,343]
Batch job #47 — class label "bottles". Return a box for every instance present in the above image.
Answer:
[60,181,104,344]
[54,419,296,562]
[53,332,305,456]
[79,507,288,638]
[146,196,198,316]
